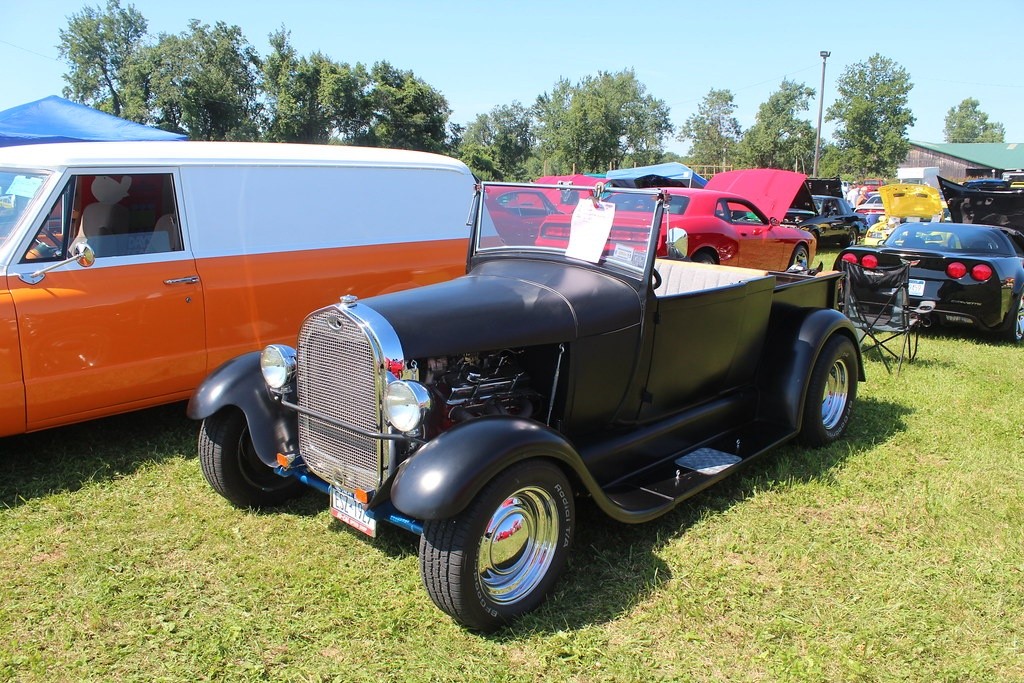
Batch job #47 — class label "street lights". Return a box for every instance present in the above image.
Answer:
[813,49,830,180]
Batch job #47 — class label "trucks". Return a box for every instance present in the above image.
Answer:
[897,167,941,191]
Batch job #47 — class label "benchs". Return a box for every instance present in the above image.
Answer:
[606,256,768,297]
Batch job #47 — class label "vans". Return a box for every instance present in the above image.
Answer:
[1,140,502,438]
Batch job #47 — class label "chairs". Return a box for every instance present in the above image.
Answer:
[902,237,993,252]
[145,214,181,252]
[68,202,147,256]
[840,258,924,376]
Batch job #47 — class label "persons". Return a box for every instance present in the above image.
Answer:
[847,181,870,212]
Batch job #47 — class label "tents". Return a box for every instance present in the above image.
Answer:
[0,96,189,148]
[606,162,708,188]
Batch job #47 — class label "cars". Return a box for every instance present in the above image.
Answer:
[533,169,818,284]
[840,178,950,250]
[778,196,870,252]
[839,175,1024,344]
[187,180,868,636]
